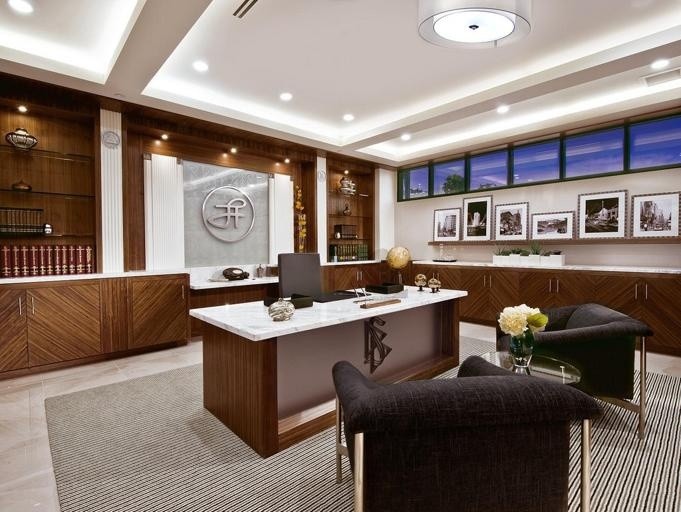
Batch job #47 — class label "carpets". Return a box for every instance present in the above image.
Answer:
[43,324,681,512]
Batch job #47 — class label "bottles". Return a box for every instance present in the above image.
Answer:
[330,245,370,260]
[343,199,353,216]
[257,264,264,278]
[440,244,444,260]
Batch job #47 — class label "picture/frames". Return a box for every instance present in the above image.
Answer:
[578,188,629,238]
[531,210,577,241]
[495,201,530,240]
[463,194,494,241]
[433,208,463,241]
[630,191,681,239]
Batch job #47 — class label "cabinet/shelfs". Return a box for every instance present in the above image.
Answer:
[0,277,117,379]
[461,265,522,320]
[1,144,96,241]
[411,264,462,320]
[321,262,380,292]
[117,272,187,355]
[522,270,595,310]
[328,189,374,242]
[596,272,681,356]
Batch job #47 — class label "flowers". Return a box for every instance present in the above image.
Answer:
[497,303,550,337]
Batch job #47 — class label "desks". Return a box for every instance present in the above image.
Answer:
[187,282,471,458]
[476,349,581,387]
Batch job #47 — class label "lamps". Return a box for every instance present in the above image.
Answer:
[415,0,536,49]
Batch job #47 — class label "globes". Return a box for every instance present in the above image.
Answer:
[386,246,409,285]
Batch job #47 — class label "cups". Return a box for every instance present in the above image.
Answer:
[267,267,273,277]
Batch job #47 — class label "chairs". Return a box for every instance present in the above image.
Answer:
[495,301,656,440]
[330,354,609,511]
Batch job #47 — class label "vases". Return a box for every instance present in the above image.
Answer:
[510,333,537,375]
